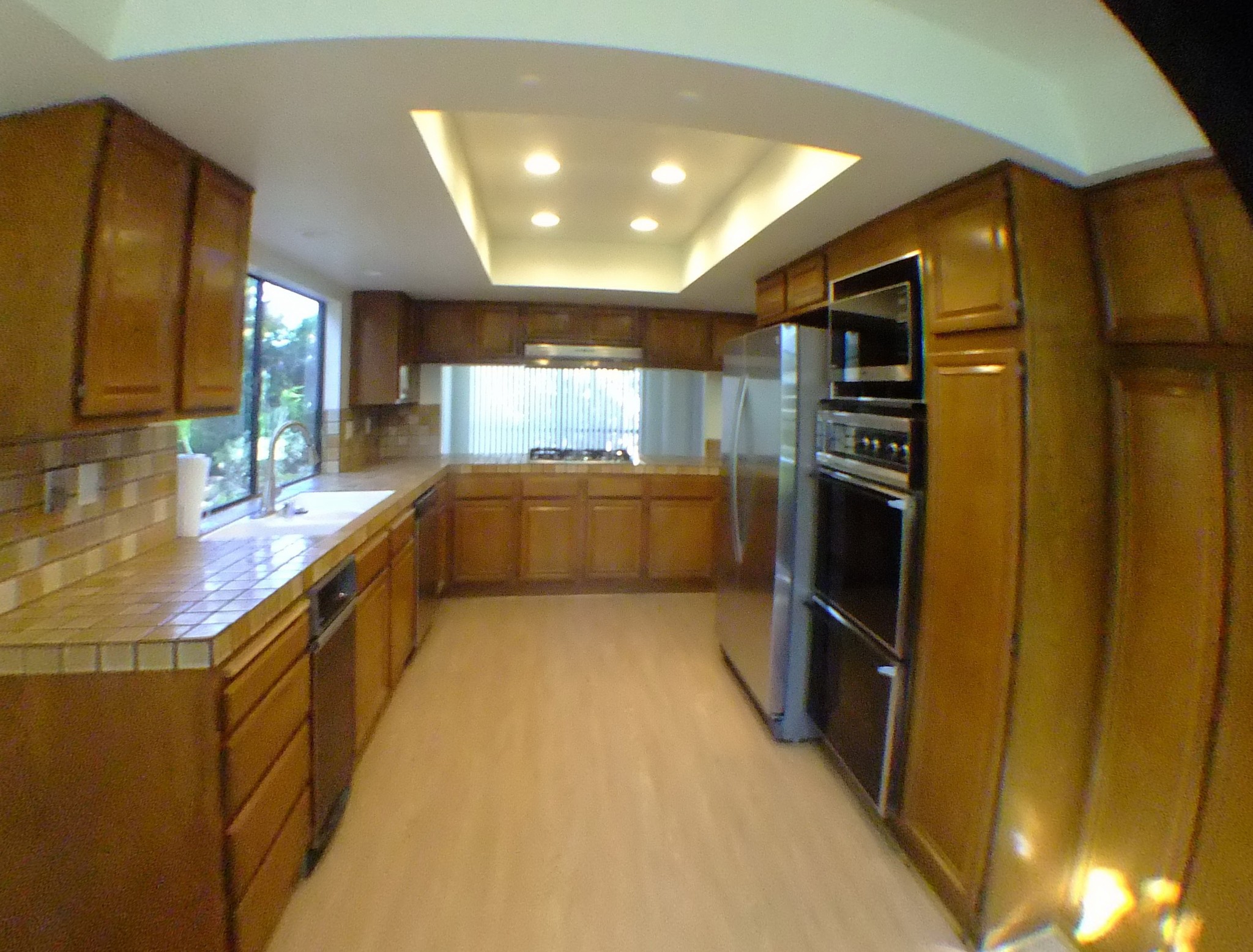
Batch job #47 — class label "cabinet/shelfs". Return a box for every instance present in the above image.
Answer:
[1,98,257,438]
[216,505,453,951]
[453,468,718,596]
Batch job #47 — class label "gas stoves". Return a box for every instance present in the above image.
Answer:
[528,448,633,465]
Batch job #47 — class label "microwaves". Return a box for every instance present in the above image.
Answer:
[829,282,922,391]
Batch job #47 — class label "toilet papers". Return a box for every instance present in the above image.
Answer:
[175,451,214,538]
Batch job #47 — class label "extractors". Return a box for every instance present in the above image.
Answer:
[526,343,643,372]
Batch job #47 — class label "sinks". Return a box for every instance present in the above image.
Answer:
[198,490,398,535]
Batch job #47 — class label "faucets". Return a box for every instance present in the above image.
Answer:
[250,419,314,520]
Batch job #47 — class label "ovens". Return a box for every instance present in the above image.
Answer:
[805,394,927,825]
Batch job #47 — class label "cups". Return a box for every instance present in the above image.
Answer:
[178,453,211,537]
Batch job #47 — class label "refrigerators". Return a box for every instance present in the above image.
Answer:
[721,321,833,746]
